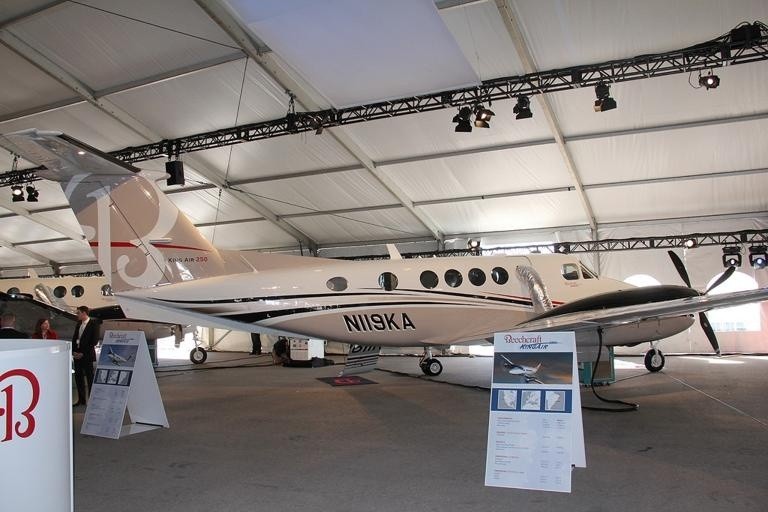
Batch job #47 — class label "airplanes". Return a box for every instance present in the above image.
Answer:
[500,355,544,384]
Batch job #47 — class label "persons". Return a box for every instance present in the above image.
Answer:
[72,306,99,407]
[32,319,58,339]
[0,311,30,338]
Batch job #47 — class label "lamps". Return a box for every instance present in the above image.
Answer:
[163,138,186,189]
[687,67,721,91]
[593,82,618,113]
[721,245,768,271]
[10,180,41,205]
[452,94,535,136]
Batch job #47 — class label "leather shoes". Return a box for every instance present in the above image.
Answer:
[73,401,86,407]
